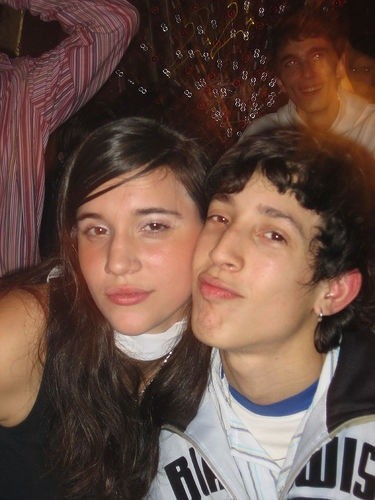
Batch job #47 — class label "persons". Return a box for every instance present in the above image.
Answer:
[237,17,375,158]
[192,133,375,500]
[0,117,208,500]
[0,0,139,280]
